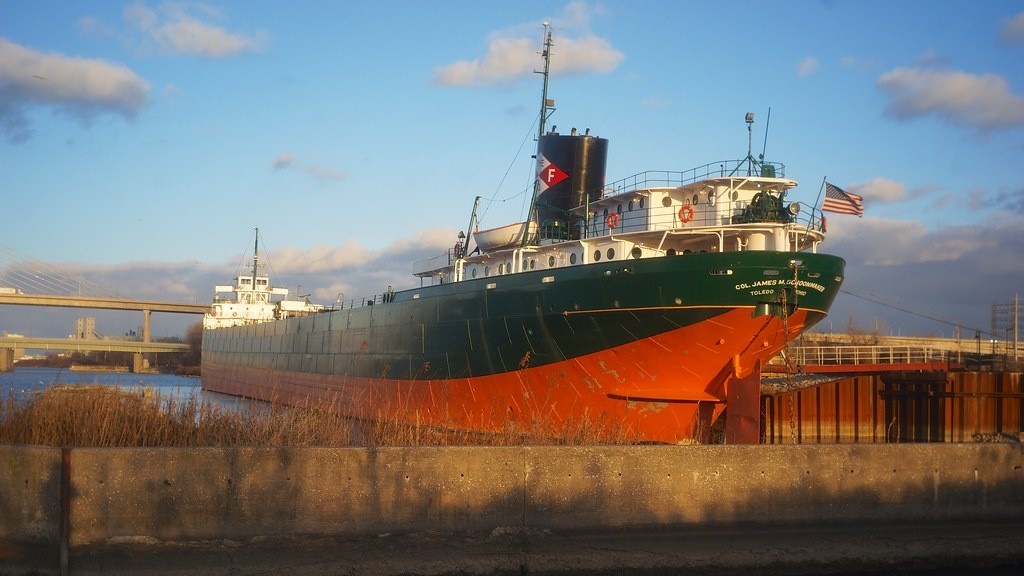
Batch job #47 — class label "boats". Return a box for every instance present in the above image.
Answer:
[199,14,847,447]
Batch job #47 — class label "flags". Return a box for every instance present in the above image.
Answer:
[820,181,863,218]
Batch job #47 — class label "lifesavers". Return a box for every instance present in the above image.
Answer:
[606,212,619,228]
[678,206,694,223]
